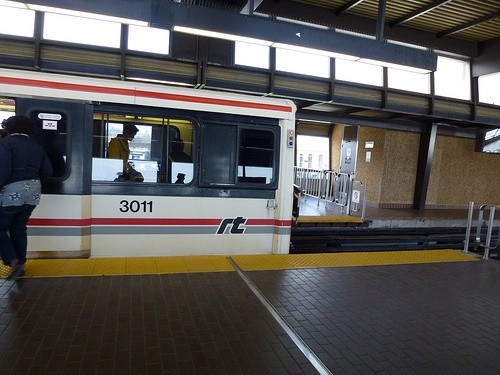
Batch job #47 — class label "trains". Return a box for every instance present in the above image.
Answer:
[1,61,298,258]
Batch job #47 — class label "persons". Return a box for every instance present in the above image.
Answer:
[114,162,144,182]
[175,173,186,184]
[108,124,139,159]
[0,115,54,280]
